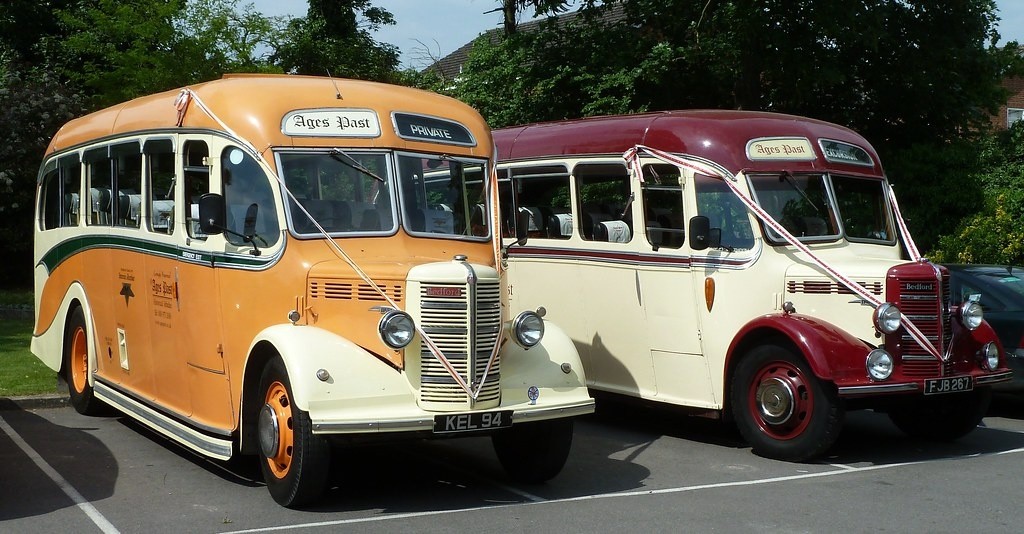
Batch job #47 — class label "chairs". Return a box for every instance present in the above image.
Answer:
[63,188,453,249]
[473,202,828,249]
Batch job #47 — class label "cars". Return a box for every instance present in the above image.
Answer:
[934,263,1024,389]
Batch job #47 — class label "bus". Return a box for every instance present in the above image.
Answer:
[30,73,595,511]
[488,110,1008,467]
